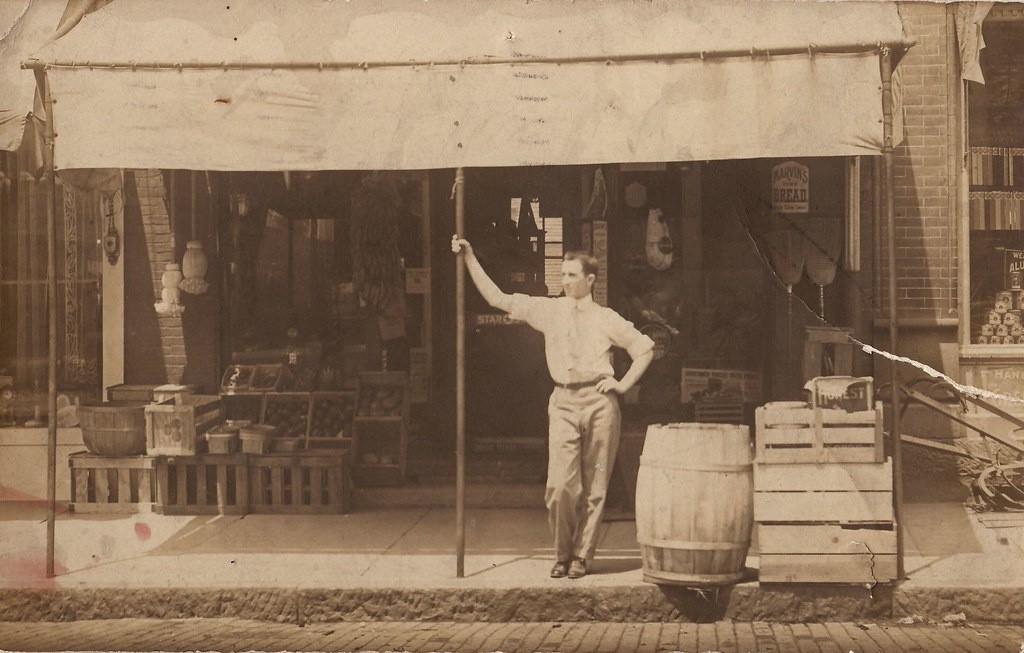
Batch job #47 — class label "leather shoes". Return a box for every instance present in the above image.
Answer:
[568,556,586,578]
[551,560,568,576]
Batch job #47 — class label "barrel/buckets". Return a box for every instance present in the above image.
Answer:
[634,423,754,587]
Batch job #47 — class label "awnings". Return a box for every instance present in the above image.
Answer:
[22,0,918,577]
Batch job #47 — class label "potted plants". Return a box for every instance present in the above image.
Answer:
[618,401,695,513]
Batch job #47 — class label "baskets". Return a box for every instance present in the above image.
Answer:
[77,401,158,457]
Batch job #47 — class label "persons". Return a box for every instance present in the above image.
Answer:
[451,234,656,579]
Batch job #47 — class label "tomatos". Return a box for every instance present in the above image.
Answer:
[264,384,404,438]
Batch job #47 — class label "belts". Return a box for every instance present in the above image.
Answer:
[555,381,599,392]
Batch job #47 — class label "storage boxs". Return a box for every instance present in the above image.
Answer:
[66,364,412,513]
